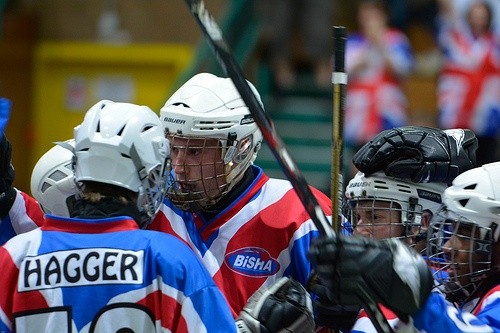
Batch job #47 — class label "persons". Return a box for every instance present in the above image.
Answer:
[0,100,238,333]
[337,0,411,146]
[435,1,500,136]
[155,73,352,333]
[249,161,500,333]
[343,128,480,262]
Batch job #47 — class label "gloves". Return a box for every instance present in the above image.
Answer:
[306,237,434,333]
[352,125,478,184]
[236,277,315,333]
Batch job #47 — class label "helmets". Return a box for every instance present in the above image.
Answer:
[31,138,79,218]
[426,162,500,301]
[52,99,172,228]
[343,171,448,258]
[160,73,264,212]
